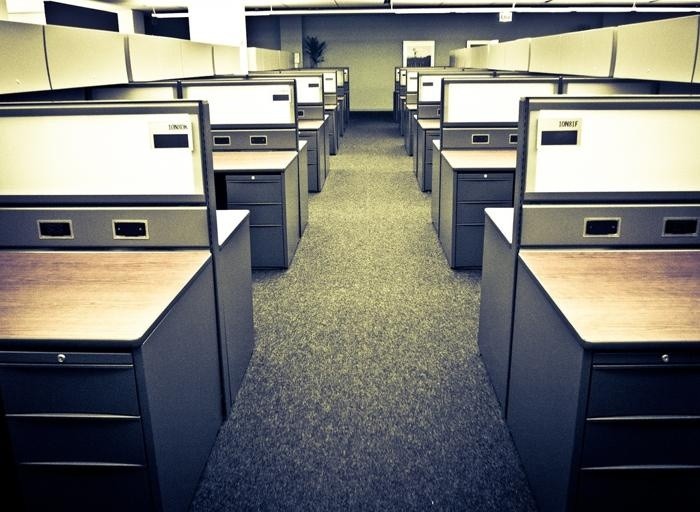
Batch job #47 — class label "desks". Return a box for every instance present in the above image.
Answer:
[299,63,350,237]
[503,248,699,509]
[440,148,514,268]
[211,151,300,270]
[478,207,515,418]
[393,65,439,238]
[214,209,255,405]
[1,248,224,510]
